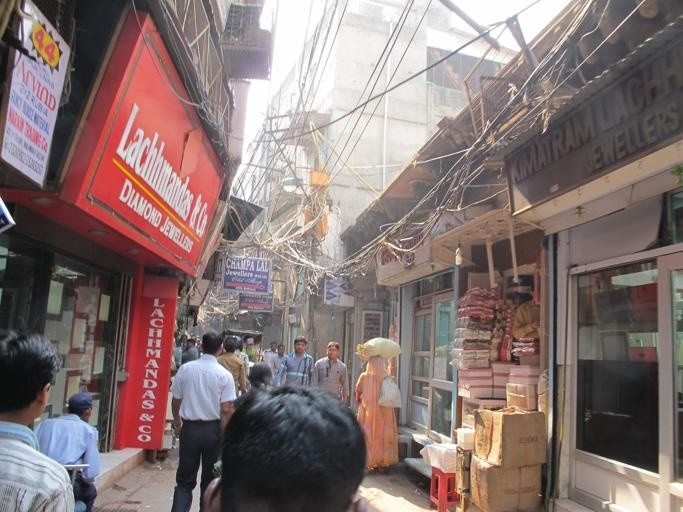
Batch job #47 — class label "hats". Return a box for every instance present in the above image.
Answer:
[69,391,93,411]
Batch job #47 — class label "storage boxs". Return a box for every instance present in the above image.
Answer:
[576,259,657,362]
[453,350,548,512]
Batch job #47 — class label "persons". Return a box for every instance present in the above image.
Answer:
[512,284,540,339]
[0,328,75,512]
[33,391,100,512]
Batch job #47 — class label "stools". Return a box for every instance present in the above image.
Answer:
[428,467,457,512]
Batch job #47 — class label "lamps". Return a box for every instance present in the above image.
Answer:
[453,239,464,266]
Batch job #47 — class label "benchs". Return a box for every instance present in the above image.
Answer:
[404,456,432,494]
